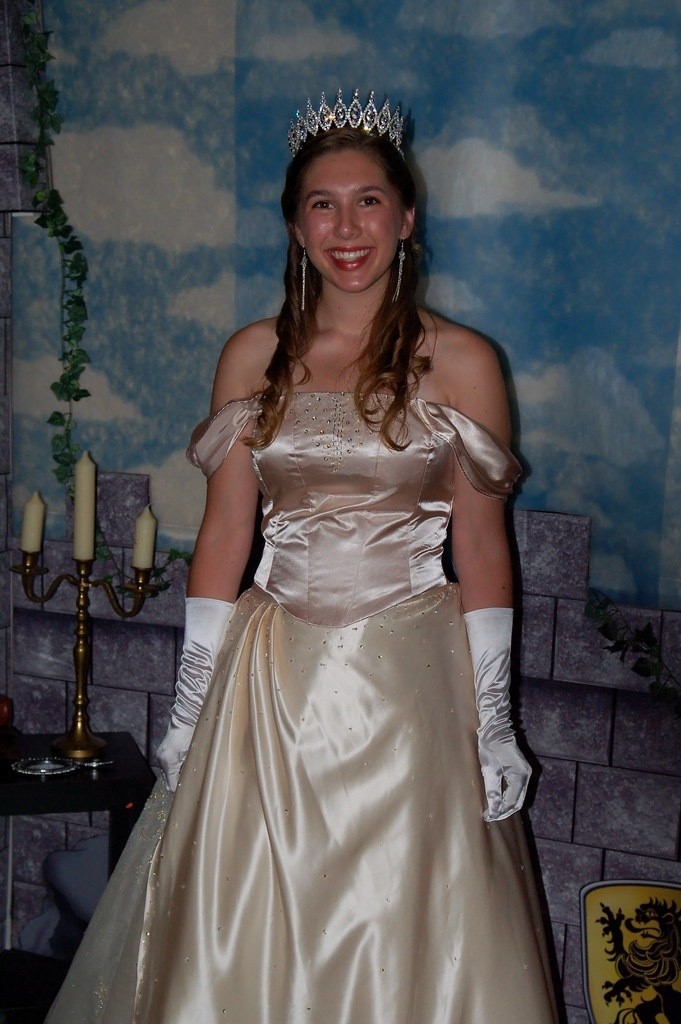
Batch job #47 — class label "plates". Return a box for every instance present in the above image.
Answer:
[14,758,77,776]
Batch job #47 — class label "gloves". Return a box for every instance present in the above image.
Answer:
[463,607,533,822]
[155,597,234,792]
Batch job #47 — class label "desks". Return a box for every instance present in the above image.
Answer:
[0,731,157,950]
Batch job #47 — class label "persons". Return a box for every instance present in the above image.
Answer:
[44,89,562,1024]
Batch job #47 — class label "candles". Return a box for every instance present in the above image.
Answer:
[73,450,95,560]
[21,491,45,553]
[132,503,156,570]
